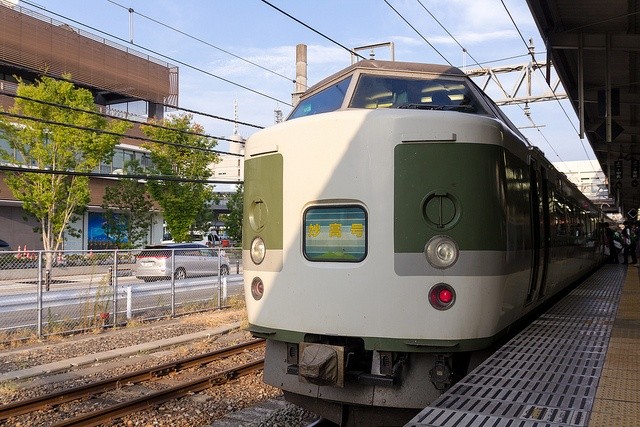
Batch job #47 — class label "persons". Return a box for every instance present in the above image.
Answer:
[629,221,640,265]
[603,222,619,264]
[619,221,637,264]
[590,222,602,256]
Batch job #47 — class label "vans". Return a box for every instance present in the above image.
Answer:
[135,243,230,282]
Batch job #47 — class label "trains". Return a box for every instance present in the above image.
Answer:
[241,59,622,427]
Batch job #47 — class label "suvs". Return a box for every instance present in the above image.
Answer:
[161,230,215,247]
